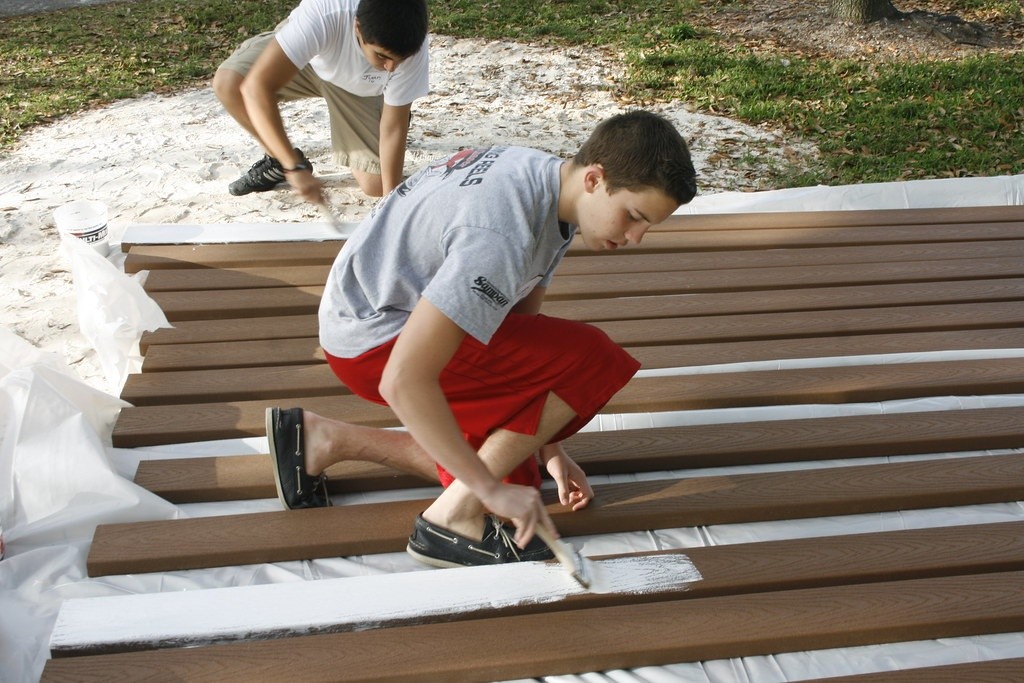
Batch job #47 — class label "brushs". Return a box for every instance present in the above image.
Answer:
[317,200,345,235]
[534,523,611,593]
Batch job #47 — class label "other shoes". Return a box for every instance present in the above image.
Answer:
[266,408,333,510]
[406,512,558,569]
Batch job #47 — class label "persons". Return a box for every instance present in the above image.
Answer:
[211,0,430,205]
[264,107,697,568]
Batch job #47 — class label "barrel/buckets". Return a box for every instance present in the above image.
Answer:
[53,199,110,259]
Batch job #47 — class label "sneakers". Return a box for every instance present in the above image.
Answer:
[229,147,313,196]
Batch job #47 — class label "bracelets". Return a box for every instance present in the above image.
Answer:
[281,160,307,173]
